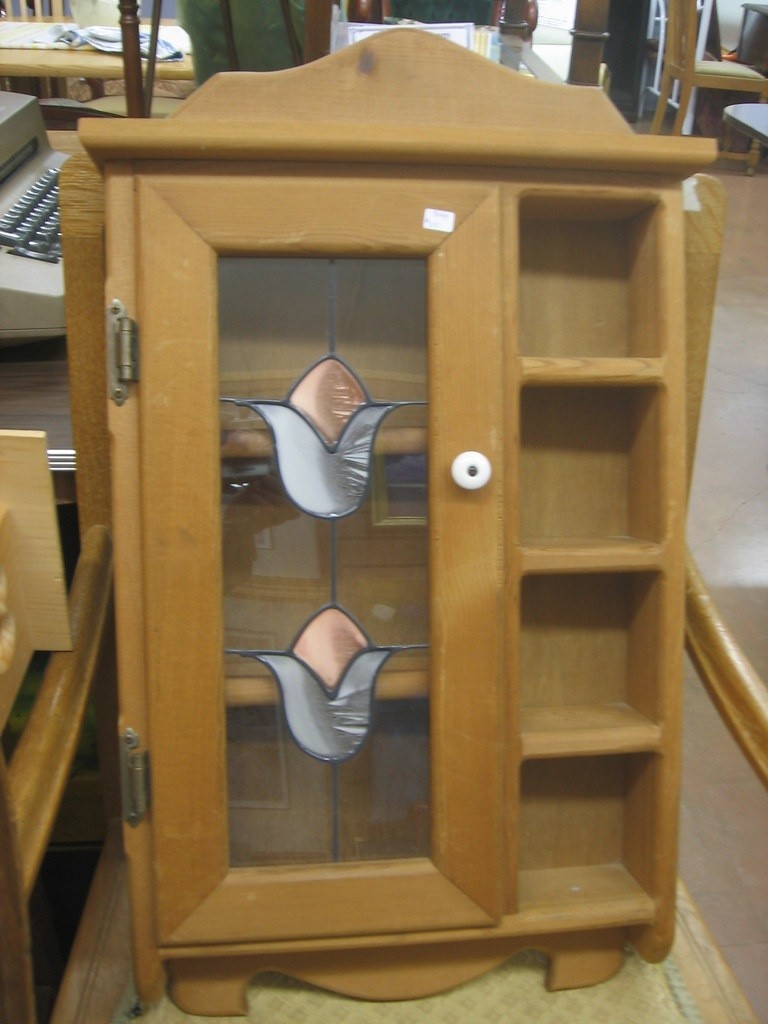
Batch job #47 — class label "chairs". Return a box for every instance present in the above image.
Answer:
[649,0,766,175]
[1,1,541,119]
[1,28,767,1023]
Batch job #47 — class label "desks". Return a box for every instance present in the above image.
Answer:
[332,22,562,82]
[0,48,196,124]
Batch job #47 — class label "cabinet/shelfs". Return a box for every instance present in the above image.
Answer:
[80,115,720,1016]
